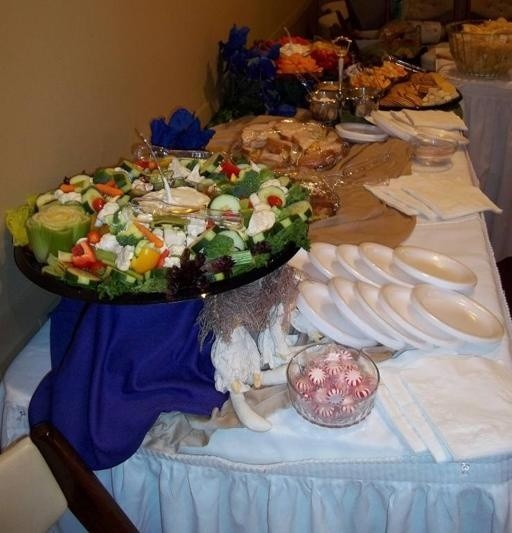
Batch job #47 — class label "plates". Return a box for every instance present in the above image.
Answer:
[14,172,308,305]
[335,121,385,145]
[343,54,463,110]
[287,241,505,352]
[370,113,472,151]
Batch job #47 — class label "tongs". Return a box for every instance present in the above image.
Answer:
[387,55,430,74]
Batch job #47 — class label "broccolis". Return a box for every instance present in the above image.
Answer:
[233,168,271,201]
[115,221,145,248]
[204,234,255,266]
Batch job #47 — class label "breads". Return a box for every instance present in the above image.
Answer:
[242,117,352,171]
[351,55,460,107]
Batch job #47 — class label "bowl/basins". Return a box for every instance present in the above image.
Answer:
[286,341,381,429]
[444,19,512,79]
[408,132,460,167]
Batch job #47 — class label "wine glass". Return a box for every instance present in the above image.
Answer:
[315,80,343,93]
[307,93,336,121]
[345,86,380,117]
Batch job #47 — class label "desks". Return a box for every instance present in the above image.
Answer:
[435,40,512,264]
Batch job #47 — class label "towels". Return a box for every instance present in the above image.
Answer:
[371,108,471,148]
[363,170,502,220]
[374,350,512,463]
